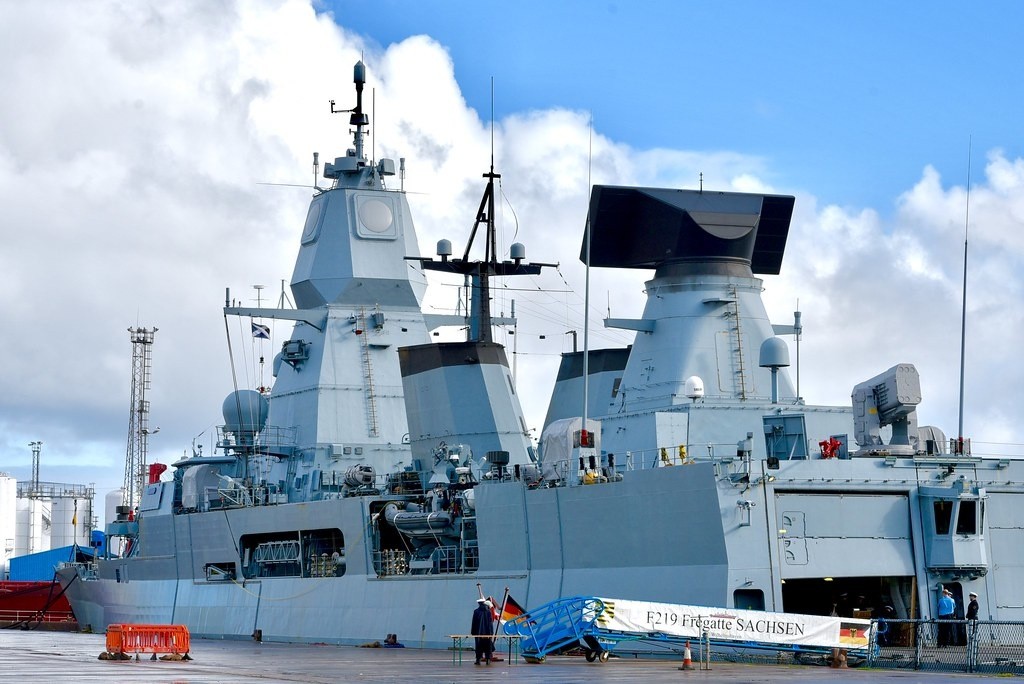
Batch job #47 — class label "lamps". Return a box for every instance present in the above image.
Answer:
[766,472,775,482]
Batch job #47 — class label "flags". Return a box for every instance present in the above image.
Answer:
[501,593,537,627]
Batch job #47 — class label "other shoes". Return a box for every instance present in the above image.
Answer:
[486,660,490,664]
[475,659,480,665]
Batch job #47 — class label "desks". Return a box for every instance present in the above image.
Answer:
[448,634,529,666]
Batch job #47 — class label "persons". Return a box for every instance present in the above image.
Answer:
[966,592,979,651]
[471,596,504,665]
[937,589,958,648]
[307,552,342,578]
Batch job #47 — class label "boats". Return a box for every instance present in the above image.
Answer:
[0,52,1023,657]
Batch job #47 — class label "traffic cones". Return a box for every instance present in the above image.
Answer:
[677,641,695,670]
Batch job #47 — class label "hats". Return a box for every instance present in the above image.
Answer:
[969,592,978,598]
[477,599,485,604]
